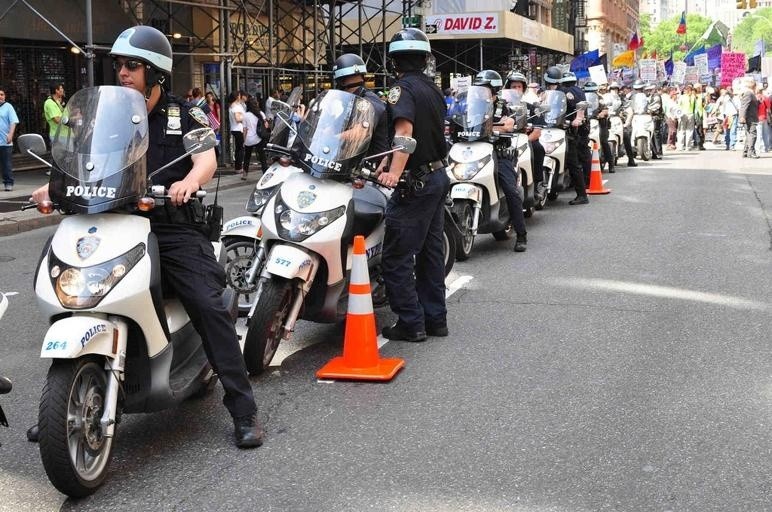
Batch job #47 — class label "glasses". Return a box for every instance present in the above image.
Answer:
[111,59,145,72]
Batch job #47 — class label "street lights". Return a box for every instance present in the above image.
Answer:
[742,16,771,32]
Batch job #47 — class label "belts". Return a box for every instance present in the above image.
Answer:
[420,159,444,176]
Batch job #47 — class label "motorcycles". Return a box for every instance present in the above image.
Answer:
[0,288,21,457]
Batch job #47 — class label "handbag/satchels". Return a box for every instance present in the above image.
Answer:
[255,118,272,142]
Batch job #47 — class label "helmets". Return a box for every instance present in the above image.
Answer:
[474,70,503,88]
[108,25,173,74]
[544,66,577,84]
[331,53,367,81]
[632,79,656,90]
[388,27,432,58]
[584,81,599,91]
[610,81,619,90]
[505,71,528,88]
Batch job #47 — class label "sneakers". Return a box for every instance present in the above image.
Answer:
[4,183,13,191]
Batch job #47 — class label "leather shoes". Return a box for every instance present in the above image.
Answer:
[747,148,760,158]
[233,408,263,449]
[609,162,615,173]
[381,318,448,343]
[568,194,589,205]
[514,232,527,252]
[652,155,662,159]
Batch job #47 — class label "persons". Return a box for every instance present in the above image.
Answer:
[30,24,265,449]
[43,80,69,145]
[0,90,20,191]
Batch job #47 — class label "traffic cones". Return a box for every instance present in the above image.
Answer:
[588,141,612,196]
[311,234,406,379]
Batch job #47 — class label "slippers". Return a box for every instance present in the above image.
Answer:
[627,162,637,166]
